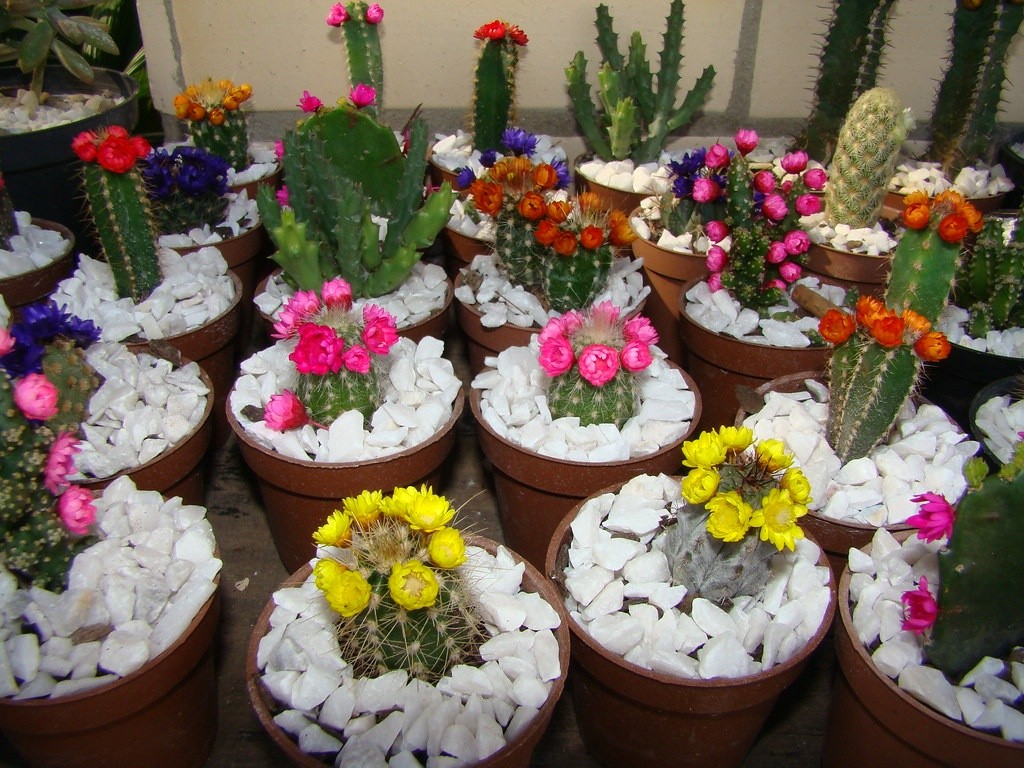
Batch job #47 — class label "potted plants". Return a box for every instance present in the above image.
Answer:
[774,0,903,201]
[0,0,139,258]
[917,197,1024,424]
[565,0,718,215]
[880,0,1024,220]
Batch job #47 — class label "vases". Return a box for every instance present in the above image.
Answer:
[0,138,1024,768]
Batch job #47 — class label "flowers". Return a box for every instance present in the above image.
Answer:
[662,425,813,606]
[466,20,529,154]
[537,300,660,432]
[327,0,384,122]
[136,144,232,236]
[468,156,638,314]
[803,187,985,467]
[173,77,255,173]
[0,297,102,440]
[263,277,399,433]
[71,125,165,306]
[0,327,99,593]
[310,483,492,686]
[256,82,459,301]
[0,173,19,252]
[824,86,917,229]
[693,129,822,346]
[642,147,765,241]
[457,124,574,225]
[899,431,1024,678]
